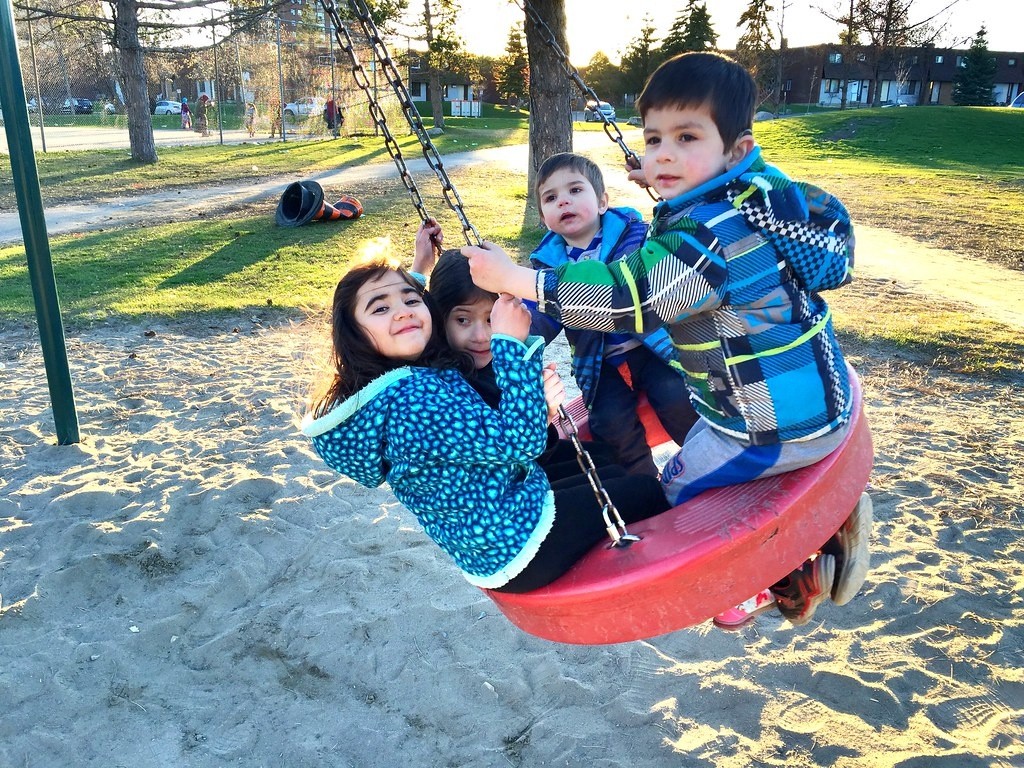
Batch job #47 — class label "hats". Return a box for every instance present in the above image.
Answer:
[182,97,188,103]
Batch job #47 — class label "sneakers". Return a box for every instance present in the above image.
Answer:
[820,492,873,605]
[712,589,777,632]
[767,554,835,627]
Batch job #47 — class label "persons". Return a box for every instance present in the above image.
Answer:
[267,93,287,138]
[301,52,874,631]
[181,98,194,130]
[243,102,257,138]
[149,94,157,115]
[194,95,212,137]
[104,98,119,115]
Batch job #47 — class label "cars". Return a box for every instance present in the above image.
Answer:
[1007,92,1024,108]
[28,95,52,114]
[584,100,616,123]
[105,97,126,115]
[153,100,182,116]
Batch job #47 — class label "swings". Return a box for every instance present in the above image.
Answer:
[315,0,877,649]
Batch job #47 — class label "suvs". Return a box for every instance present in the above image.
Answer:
[284,96,346,124]
[59,98,94,115]
